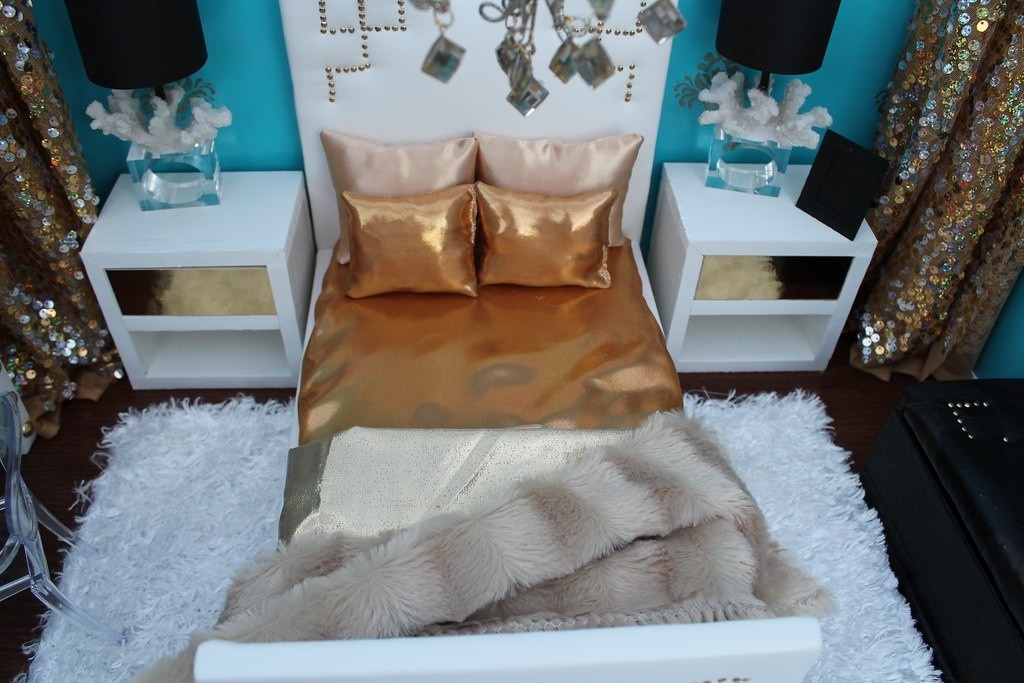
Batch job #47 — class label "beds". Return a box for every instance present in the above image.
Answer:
[193,0,822,683]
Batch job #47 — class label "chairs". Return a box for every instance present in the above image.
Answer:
[0,360,127,647]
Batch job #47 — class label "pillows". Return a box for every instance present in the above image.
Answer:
[320,129,479,264]
[472,129,643,247]
[341,182,478,298]
[476,182,618,289]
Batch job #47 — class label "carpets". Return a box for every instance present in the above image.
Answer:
[30,392,944,683]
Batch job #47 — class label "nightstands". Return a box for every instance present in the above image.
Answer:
[646,161,878,372]
[79,171,315,393]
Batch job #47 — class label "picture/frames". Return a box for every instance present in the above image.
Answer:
[796,130,889,240]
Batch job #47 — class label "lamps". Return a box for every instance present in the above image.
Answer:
[715,0,841,96]
[63,0,208,101]
